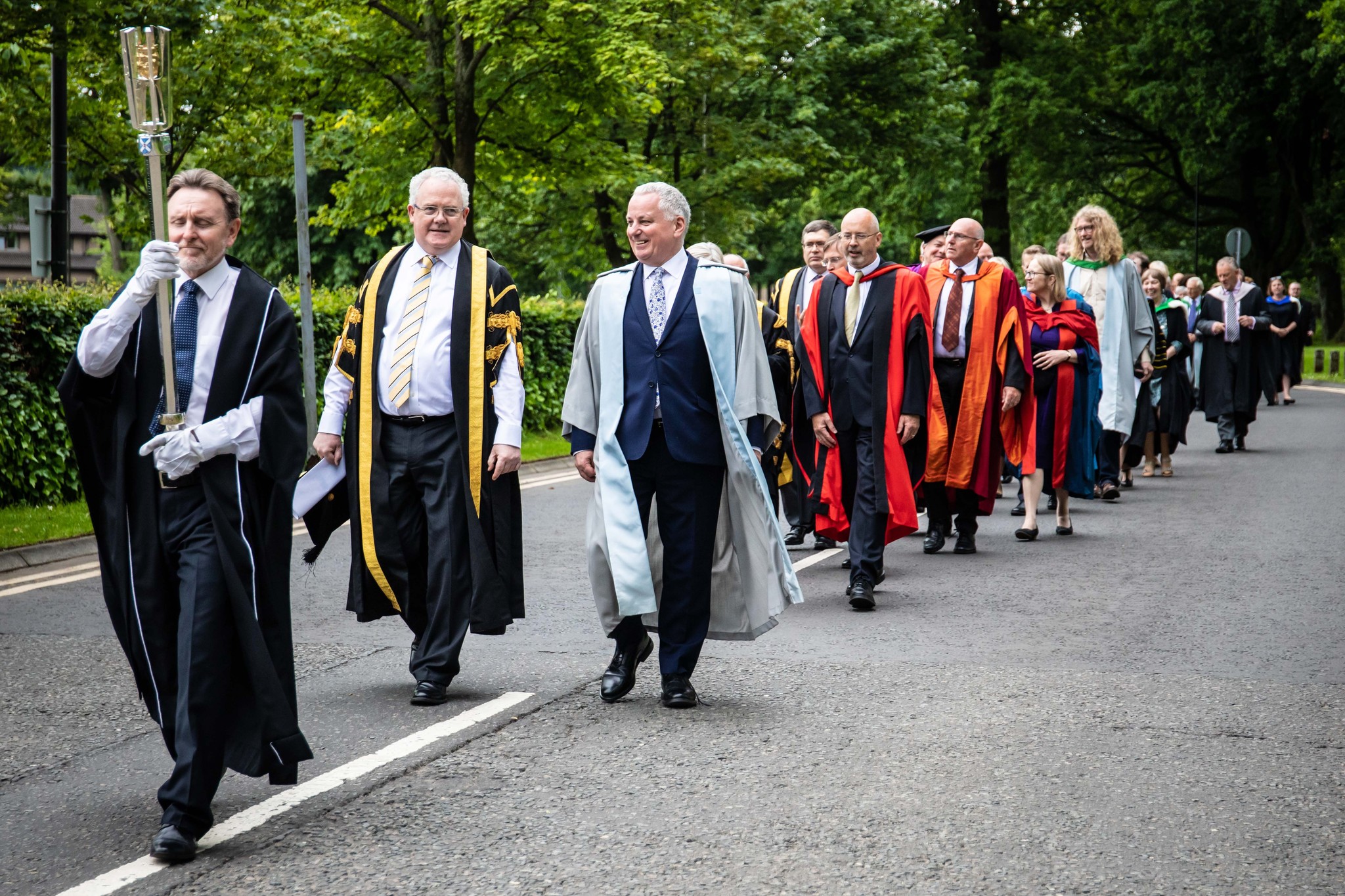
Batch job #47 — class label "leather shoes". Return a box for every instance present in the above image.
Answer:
[783,528,837,550]
[1048,495,1057,509]
[599,627,654,702]
[842,555,885,608]
[661,673,714,708]
[1011,501,1038,516]
[150,823,197,862]
[410,680,448,704]
[923,530,977,554]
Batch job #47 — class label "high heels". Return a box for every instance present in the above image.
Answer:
[1015,524,1039,541]
[1142,457,1173,477]
[1056,517,1073,535]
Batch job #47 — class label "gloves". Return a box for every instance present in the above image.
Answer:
[139,423,206,480]
[126,239,181,310]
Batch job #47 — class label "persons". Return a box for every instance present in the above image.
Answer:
[1117,252,1204,487]
[1210,276,1318,405]
[793,208,932,607]
[686,219,848,550]
[560,182,804,706]
[1061,204,1155,499]
[917,218,1033,555]
[57,168,314,860]
[905,225,1073,295]
[1196,256,1274,453]
[1011,245,1103,540]
[314,167,525,705]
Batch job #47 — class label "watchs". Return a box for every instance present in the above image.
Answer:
[1065,349,1072,362]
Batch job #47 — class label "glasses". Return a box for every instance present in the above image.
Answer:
[820,256,845,267]
[838,232,878,241]
[944,231,980,241]
[1270,276,1281,282]
[1074,225,1094,234]
[801,242,824,251]
[1025,270,1051,279]
[414,204,464,218]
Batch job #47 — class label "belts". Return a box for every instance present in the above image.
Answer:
[383,413,455,425]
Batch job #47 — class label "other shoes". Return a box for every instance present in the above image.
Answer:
[996,484,1003,498]
[1093,469,1133,500]
[1283,399,1295,406]
[1216,436,1245,453]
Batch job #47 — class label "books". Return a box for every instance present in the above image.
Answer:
[291,441,346,519]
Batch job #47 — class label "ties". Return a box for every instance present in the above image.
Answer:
[815,276,822,280]
[148,279,201,438]
[648,268,665,409]
[1227,291,1239,344]
[388,255,440,409]
[1187,300,1197,333]
[844,271,864,347]
[941,268,965,353]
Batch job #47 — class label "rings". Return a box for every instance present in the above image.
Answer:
[912,435,915,437]
